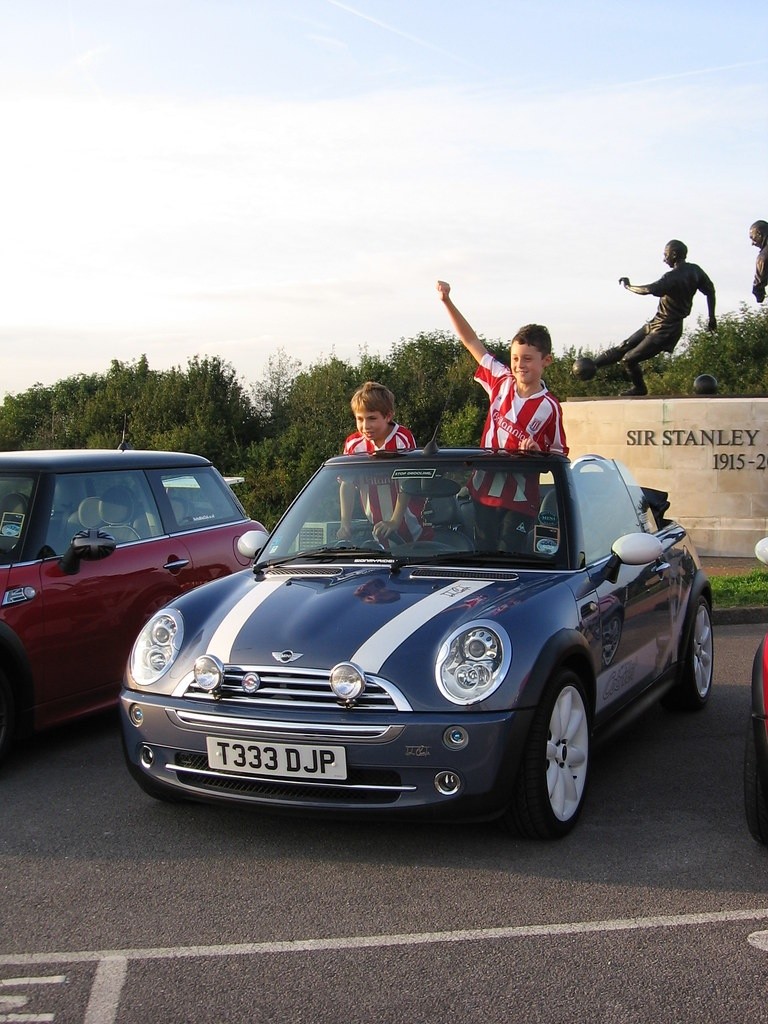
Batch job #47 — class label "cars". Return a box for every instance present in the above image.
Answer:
[126,444,715,843]
[0,451,269,756]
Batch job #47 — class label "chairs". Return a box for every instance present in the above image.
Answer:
[134,499,199,538]
[425,493,473,550]
[527,490,561,555]
[99,487,139,545]
[78,496,104,530]
[3,493,30,528]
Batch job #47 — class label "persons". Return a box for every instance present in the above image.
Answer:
[749,220,768,303]
[436,280,569,551]
[335,381,434,550]
[571,239,717,396]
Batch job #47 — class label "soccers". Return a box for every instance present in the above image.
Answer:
[692,374,717,395]
[571,358,597,381]
